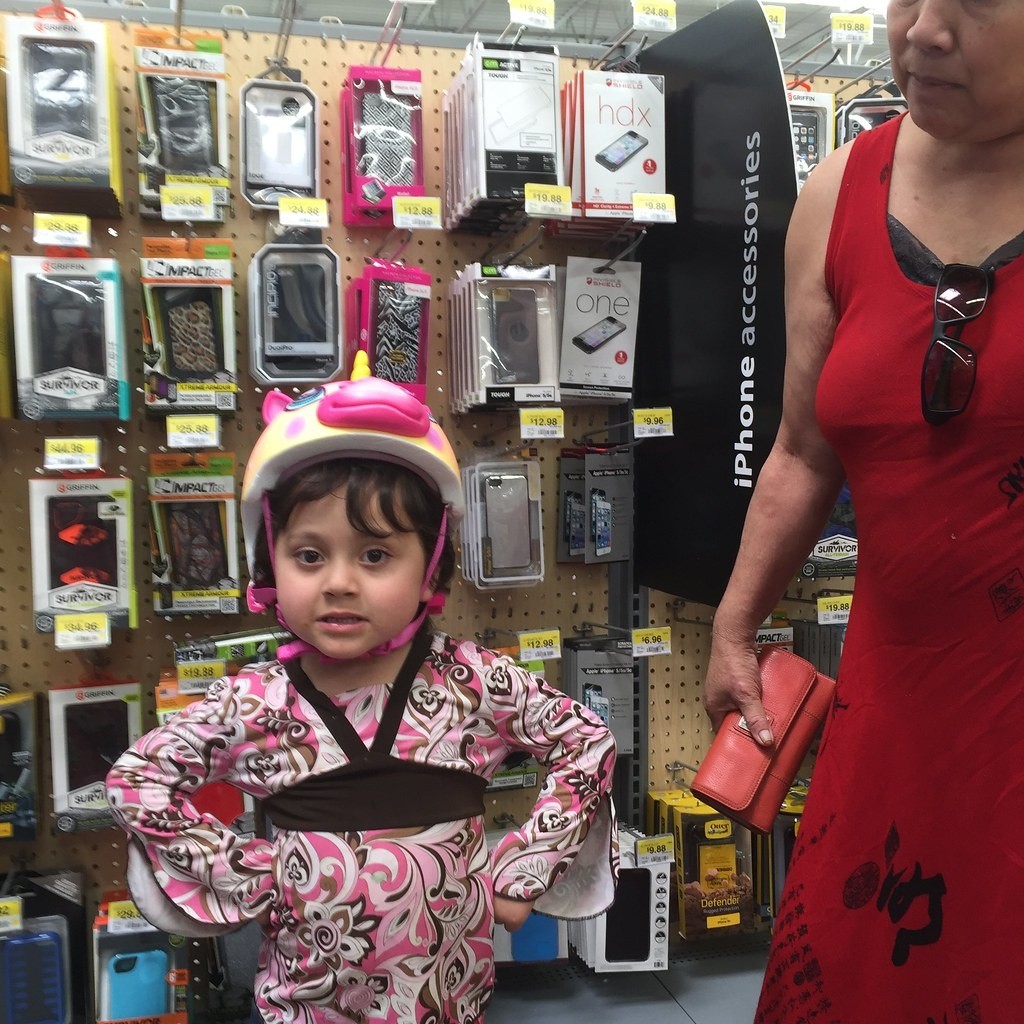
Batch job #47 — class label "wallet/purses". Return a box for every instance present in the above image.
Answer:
[699,646,841,838]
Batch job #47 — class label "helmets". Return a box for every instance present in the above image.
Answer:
[240,376,464,567]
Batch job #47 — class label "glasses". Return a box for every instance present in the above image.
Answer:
[923,259,991,430]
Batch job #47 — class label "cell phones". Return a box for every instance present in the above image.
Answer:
[595,131,648,173]
[606,867,652,963]
[791,110,820,179]
[564,488,612,557]
[572,316,626,355]
[582,682,610,728]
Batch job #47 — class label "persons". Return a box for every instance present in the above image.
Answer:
[698,3,1024,1023]
[105,372,618,1024]
